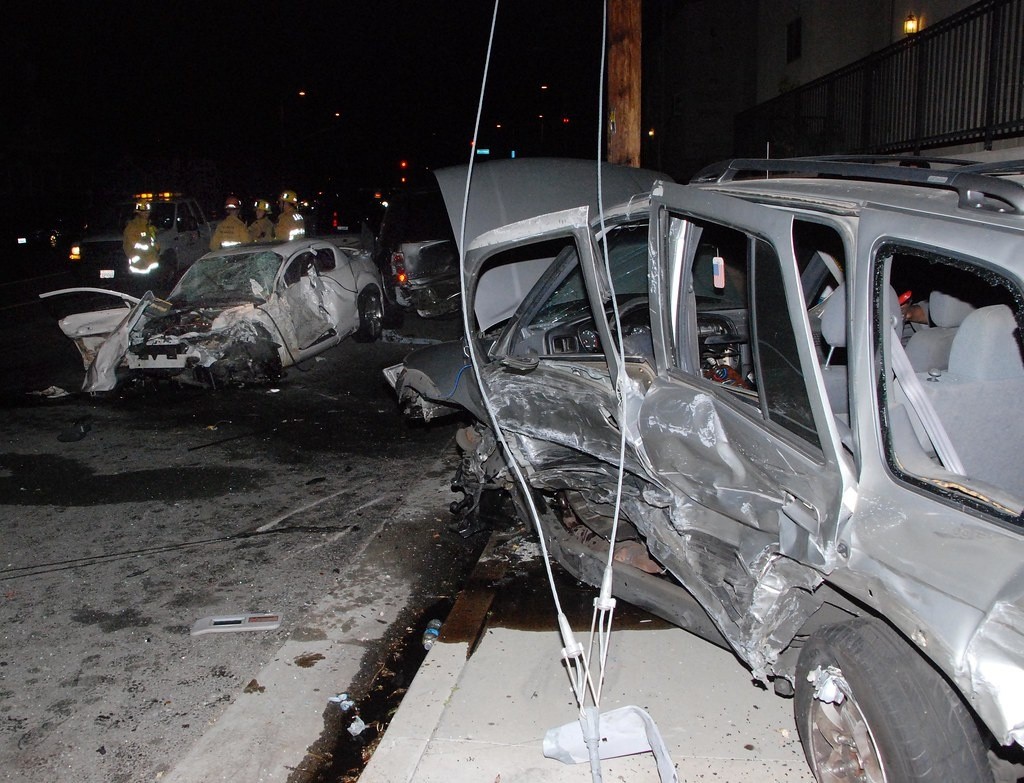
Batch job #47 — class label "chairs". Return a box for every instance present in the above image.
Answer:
[894,304,1024,499]
[817,282,903,414]
[904,266,976,375]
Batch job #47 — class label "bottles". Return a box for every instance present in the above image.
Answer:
[421,619,442,650]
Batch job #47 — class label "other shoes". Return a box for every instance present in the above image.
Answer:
[57,425,91,442]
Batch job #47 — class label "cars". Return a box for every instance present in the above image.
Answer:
[0,191,462,405]
[427,152,1023,783]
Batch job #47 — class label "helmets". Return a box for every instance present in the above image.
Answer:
[253,200,271,212]
[133,199,152,213]
[224,198,242,209]
[278,190,297,204]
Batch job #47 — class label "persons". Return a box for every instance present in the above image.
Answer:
[893,275,931,348]
[247,198,274,239]
[123,201,160,297]
[275,191,305,240]
[209,197,249,252]
